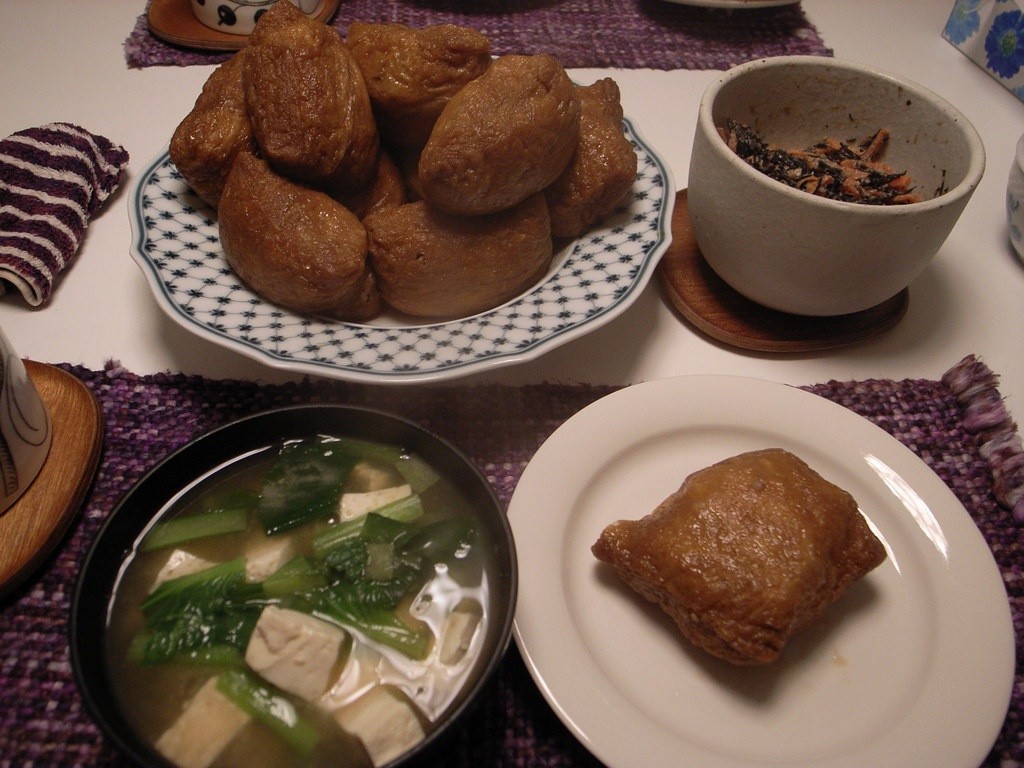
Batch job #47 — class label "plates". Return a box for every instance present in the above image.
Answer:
[657,188,910,354]
[129,114,677,387]
[0,358,105,604]
[506,374,1016,768]
[146,0,340,52]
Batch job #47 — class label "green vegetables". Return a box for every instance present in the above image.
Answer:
[728,118,921,209]
[126,439,489,755]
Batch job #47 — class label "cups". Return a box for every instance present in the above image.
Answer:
[0,329,54,517]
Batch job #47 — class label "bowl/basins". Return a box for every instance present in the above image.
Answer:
[191,0,328,36]
[65,403,520,768]
[688,55,988,319]
